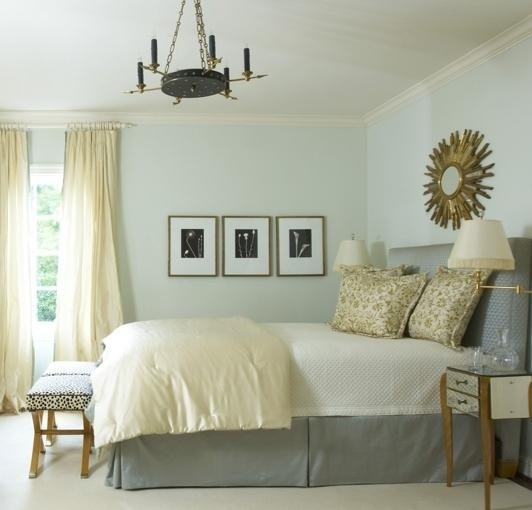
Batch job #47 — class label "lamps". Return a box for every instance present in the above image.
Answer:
[446,222,532,294]
[120,0,269,106]
[333,234,373,273]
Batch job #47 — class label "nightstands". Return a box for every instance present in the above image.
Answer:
[439,365,532,510]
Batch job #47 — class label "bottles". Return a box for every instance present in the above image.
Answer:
[486,328,519,371]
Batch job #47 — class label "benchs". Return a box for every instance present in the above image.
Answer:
[25,361,95,480]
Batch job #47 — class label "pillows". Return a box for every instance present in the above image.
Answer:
[325,265,491,352]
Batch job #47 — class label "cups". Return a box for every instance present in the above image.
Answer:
[467,346,483,372]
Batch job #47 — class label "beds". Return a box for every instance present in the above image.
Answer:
[86,238,532,488]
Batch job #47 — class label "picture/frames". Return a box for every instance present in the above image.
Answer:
[167,215,334,269]
